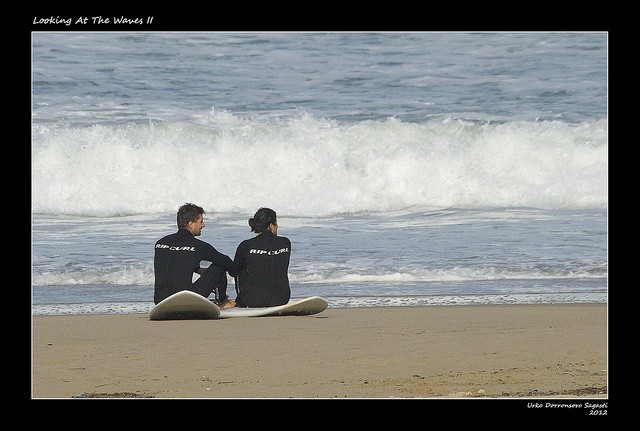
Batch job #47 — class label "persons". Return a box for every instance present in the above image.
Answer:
[154,203,241,309]
[234,208,293,307]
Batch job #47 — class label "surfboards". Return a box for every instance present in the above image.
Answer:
[217,296,328,319]
[150,289,220,320]
